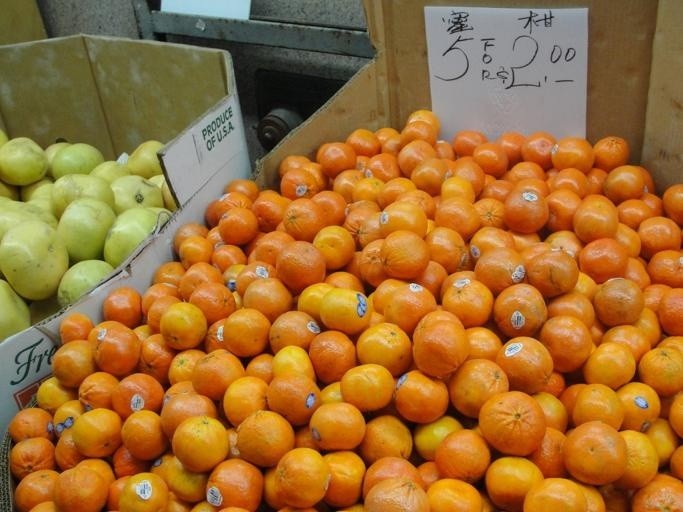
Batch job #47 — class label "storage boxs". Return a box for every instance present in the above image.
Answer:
[0,0,683,512]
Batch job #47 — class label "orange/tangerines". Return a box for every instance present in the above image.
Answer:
[0,107,683,512]
